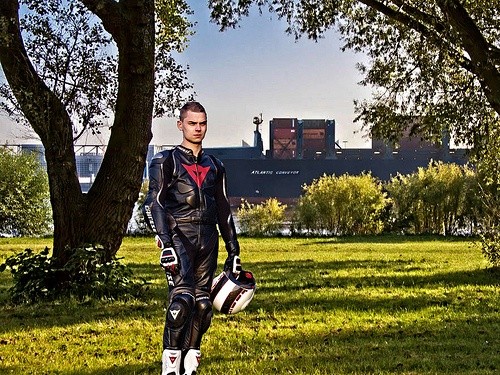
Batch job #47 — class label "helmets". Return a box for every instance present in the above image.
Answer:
[210,270,256,314]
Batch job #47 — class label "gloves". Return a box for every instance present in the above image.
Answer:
[159,242,182,277]
[223,251,242,281]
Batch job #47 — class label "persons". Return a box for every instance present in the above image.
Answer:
[143,101,241,375]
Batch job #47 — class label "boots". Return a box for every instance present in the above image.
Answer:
[160,346,182,375]
[180,347,202,375]
